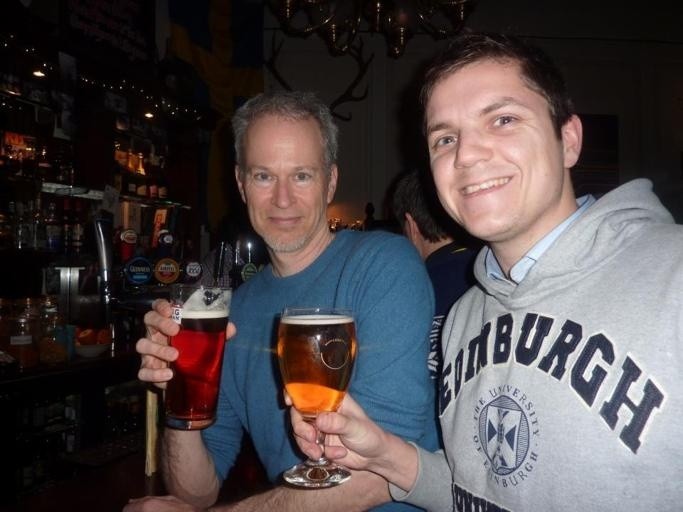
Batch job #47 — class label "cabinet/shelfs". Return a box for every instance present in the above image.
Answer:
[0,39,209,511]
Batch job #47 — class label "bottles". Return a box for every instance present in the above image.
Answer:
[0,148,168,499]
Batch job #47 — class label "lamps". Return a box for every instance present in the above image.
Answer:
[265,0,471,59]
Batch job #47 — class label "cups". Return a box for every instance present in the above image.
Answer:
[159,283,233,430]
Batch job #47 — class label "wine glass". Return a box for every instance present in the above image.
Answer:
[278,307,357,488]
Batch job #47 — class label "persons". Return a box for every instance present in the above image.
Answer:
[388,151,487,453]
[281,30,682,512]
[121,88,447,512]
